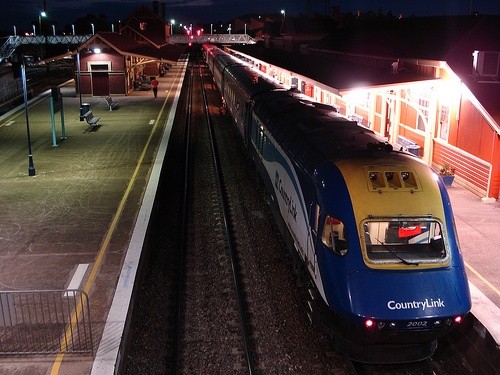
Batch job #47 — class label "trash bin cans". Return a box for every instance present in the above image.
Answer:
[160,70,164,77]
[134,81,139,89]
[406,145,420,156]
[82,104,90,118]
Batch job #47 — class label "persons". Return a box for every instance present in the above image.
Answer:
[152,77,158,98]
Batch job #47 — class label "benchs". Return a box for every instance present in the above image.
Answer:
[105,96,118,111]
[83,111,100,131]
[160,63,170,77]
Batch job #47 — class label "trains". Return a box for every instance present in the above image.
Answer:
[203,42,471,334]
